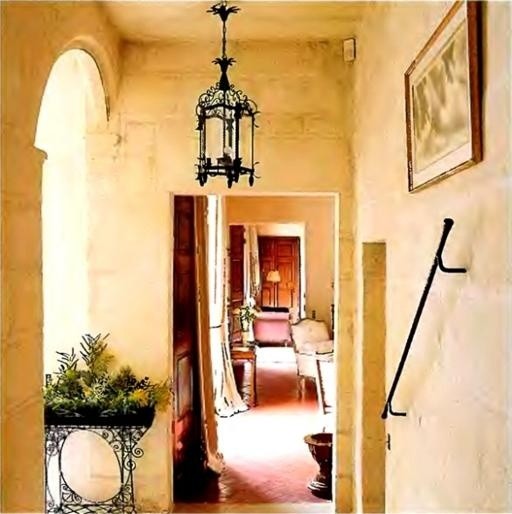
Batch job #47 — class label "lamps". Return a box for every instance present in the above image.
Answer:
[195,0,261,189]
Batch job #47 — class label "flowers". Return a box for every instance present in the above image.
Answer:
[44,333,173,413]
[232,303,261,331]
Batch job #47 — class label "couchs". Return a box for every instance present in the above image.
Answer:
[255,311,290,347]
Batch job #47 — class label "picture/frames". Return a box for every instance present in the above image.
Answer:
[403,0,483,196]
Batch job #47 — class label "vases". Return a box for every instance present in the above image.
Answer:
[241,331,250,347]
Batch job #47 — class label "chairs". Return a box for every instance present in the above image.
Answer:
[288,317,334,414]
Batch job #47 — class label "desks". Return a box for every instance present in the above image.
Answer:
[230,342,258,407]
